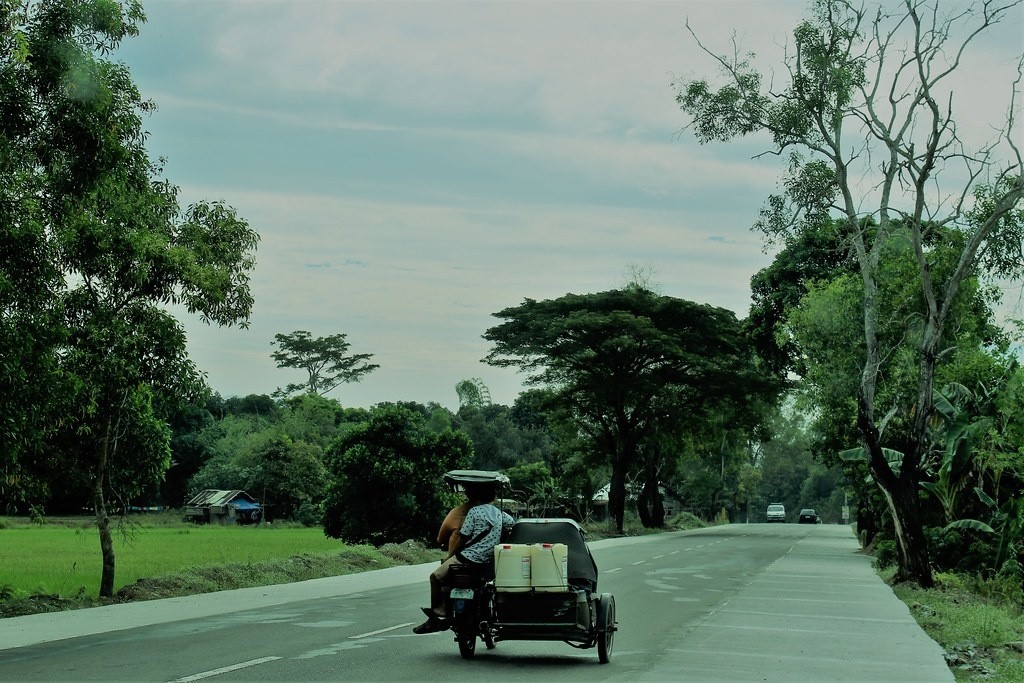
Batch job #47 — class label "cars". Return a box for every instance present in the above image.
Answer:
[799,508,822,524]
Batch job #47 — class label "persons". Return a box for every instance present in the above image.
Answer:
[251,509,260,523]
[412,482,515,634]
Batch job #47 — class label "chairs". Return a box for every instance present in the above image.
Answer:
[512,519,596,591]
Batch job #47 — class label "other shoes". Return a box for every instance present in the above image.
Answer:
[413,617,451,634]
[420,607,453,617]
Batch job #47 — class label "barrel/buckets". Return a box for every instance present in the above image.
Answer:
[494,543,532,592]
[531,542,568,592]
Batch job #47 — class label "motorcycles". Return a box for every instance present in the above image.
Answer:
[444,469,621,665]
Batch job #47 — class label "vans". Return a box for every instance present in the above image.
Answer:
[765,503,787,523]
